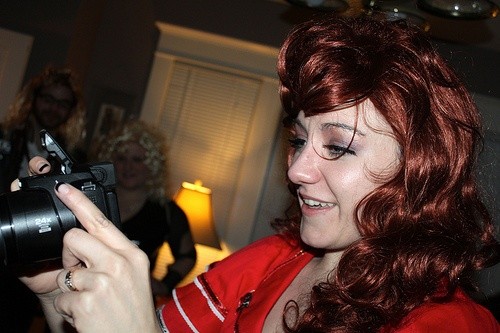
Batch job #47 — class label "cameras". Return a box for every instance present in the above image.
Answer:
[0,128,121,278]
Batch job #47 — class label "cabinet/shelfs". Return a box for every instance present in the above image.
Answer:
[138,22,292,253]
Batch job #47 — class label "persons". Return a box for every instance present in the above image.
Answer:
[12,7,500,333]
[0,68,197,310]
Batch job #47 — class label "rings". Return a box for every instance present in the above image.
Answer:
[65,266,81,291]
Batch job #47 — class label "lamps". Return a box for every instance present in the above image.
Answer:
[277,0,499,25]
[172,180,222,250]
